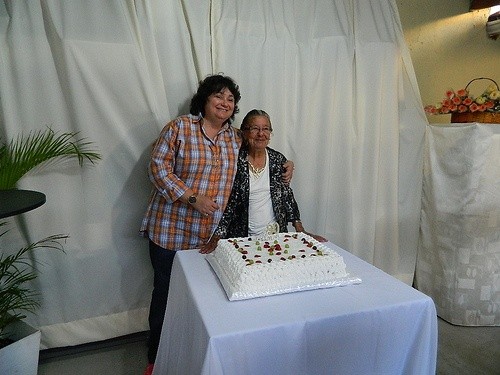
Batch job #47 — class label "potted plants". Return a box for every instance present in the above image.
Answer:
[0,122,103,375]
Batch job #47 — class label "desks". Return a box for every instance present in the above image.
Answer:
[150,240,438,375]
[0,190,46,219]
[413,123,500,326]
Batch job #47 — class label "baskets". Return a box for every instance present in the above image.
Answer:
[449,77,500,123]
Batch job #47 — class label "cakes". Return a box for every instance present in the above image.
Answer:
[214,231,347,296]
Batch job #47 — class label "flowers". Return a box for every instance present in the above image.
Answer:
[425,82,500,115]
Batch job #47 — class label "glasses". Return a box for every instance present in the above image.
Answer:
[244,126,269,134]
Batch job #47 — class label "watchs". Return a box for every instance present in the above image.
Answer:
[188,193,199,206]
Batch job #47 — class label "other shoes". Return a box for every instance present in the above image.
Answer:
[144,362,154,375]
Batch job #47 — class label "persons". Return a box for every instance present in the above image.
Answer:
[138,75,294,375]
[199,110,328,254]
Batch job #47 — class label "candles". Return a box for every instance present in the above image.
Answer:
[266,222,280,236]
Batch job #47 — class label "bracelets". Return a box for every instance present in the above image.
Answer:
[292,220,302,227]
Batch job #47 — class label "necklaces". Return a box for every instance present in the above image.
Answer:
[249,162,264,173]
